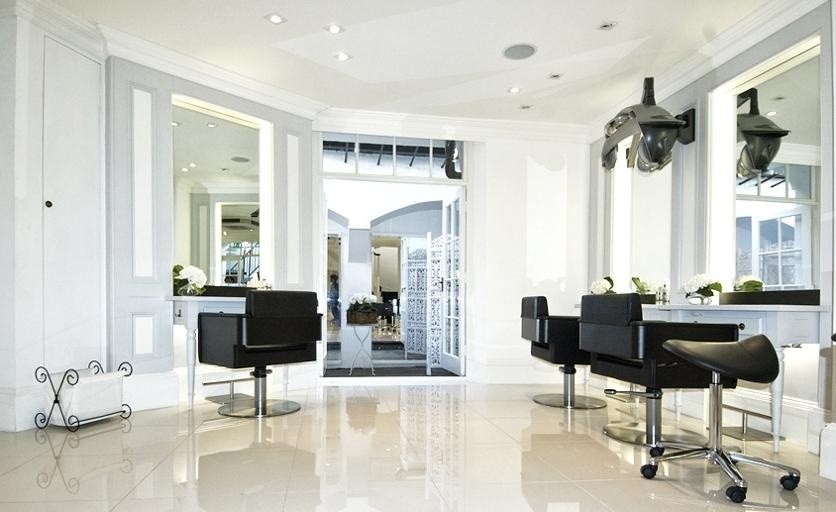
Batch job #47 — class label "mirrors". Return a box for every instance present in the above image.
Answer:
[170,91,274,296]
[600,111,684,299]
[694,29,824,294]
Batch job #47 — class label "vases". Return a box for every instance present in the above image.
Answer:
[346,311,378,324]
[687,293,713,305]
[177,282,200,295]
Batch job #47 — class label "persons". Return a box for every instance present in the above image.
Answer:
[328,274,339,323]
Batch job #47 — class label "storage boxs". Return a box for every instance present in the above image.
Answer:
[44,367,127,428]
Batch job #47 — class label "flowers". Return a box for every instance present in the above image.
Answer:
[681,271,723,297]
[349,291,377,309]
[632,273,654,295]
[174,265,207,289]
[731,273,764,292]
[589,274,617,295]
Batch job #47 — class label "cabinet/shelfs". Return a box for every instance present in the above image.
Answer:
[760,239,801,285]
[736,248,754,276]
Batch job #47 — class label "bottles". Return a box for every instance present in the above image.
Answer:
[662,284,668,304]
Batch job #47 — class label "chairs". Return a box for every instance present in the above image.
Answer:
[577,292,739,450]
[198,289,323,420]
[520,296,607,411]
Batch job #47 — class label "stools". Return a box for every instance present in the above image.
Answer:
[641,334,801,503]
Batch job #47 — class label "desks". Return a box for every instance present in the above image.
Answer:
[164,294,291,411]
[345,323,380,376]
